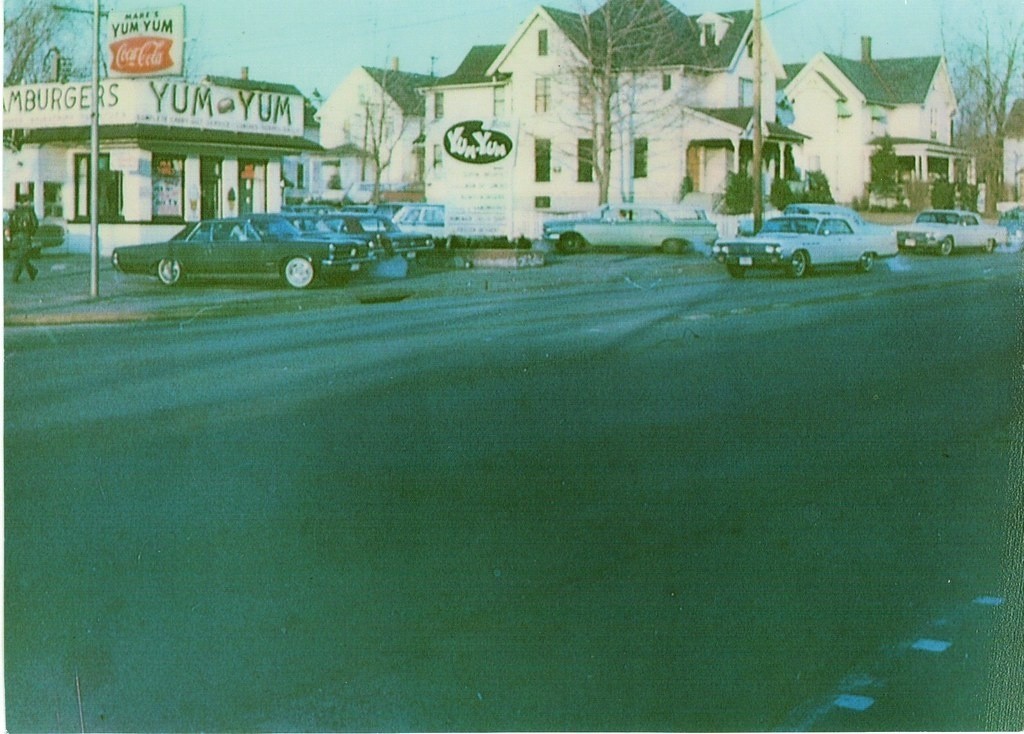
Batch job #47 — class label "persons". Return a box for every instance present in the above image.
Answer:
[11,194,39,283]
[618,210,628,222]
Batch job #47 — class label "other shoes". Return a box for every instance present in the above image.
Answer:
[30,269,38,280]
[12,276,19,282]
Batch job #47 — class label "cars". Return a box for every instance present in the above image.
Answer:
[3,206,67,259]
[110,214,379,291]
[895,209,1008,257]
[239,200,445,254]
[997,209,1024,247]
[712,203,899,278]
[538,198,720,255]
